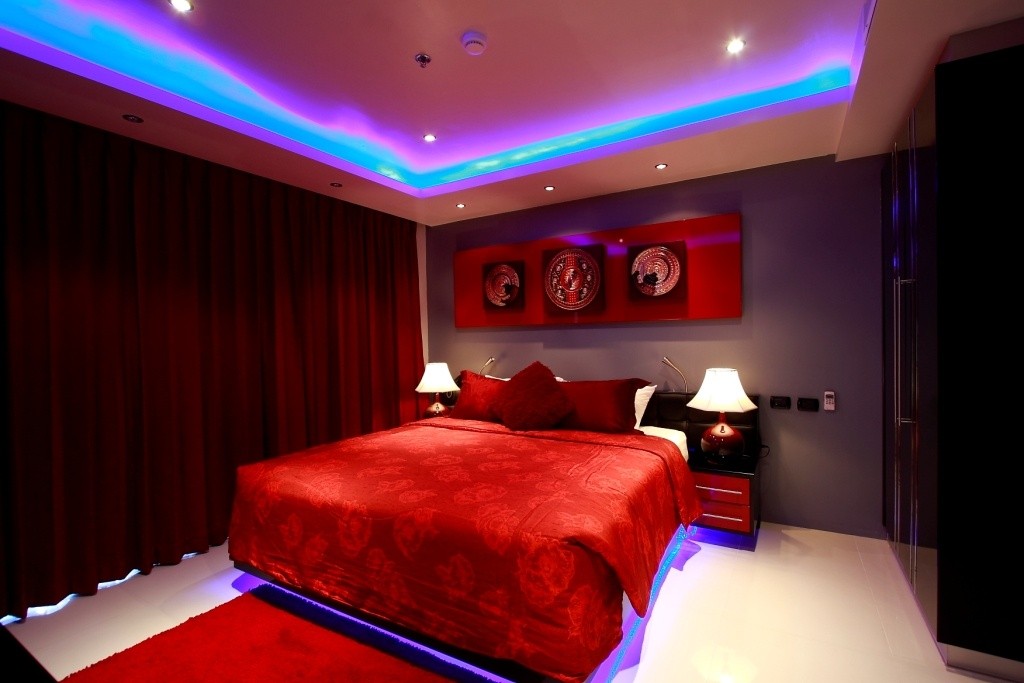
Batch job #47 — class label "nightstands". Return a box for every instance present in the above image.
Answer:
[687,451,761,536]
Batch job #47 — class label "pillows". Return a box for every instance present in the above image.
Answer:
[447,370,656,432]
[493,361,576,430]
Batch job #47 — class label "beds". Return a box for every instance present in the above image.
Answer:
[229,370,705,683]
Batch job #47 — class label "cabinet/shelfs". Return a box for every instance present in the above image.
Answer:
[878,41,1024,677]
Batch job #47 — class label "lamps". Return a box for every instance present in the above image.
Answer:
[415,362,460,418]
[686,367,758,464]
[479,356,495,375]
[662,356,688,392]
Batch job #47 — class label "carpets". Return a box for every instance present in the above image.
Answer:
[57,582,497,683]
